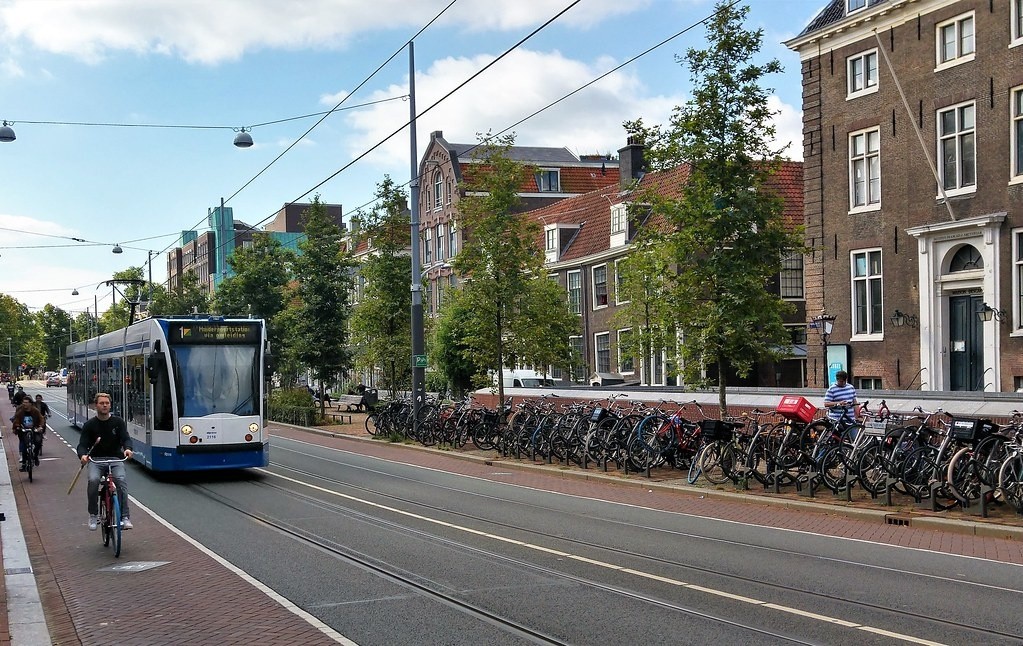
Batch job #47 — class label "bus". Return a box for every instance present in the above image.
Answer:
[67,314,274,470]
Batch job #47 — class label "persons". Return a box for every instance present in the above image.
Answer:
[77,393,133,531]
[45,373,63,388]
[0,370,2,383]
[29,370,33,380]
[6,381,26,406]
[306,384,330,401]
[348,384,371,410]
[9,394,51,471]
[814,370,864,462]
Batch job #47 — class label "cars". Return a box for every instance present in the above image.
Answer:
[44,371,58,381]
[46,376,60,388]
[0,373,16,384]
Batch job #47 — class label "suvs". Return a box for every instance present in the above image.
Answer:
[58,368,67,388]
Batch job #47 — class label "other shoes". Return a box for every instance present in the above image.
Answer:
[18,452,23,462]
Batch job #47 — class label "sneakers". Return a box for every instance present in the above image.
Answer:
[121,517,133,529]
[88,514,98,531]
[34,455,40,467]
[19,464,26,472]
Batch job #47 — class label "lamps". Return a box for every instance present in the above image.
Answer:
[71,288,79,295]
[112,244,123,255]
[232,127,254,149]
[0,120,16,143]
[891,309,918,330]
[976,300,1008,325]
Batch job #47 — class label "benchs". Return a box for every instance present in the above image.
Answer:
[333,393,363,412]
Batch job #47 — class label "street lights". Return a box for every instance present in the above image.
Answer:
[413,259,461,439]
[7,337,12,384]
[811,307,838,388]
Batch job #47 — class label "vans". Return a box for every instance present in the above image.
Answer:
[493,370,558,388]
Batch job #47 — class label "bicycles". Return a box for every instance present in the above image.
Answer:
[822,400,1023,514]
[365,389,516,453]
[13,400,52,433]
[505,393,698,472]
[87,450,134,558]
[13,428,43,483]
[687,402,840,487]
